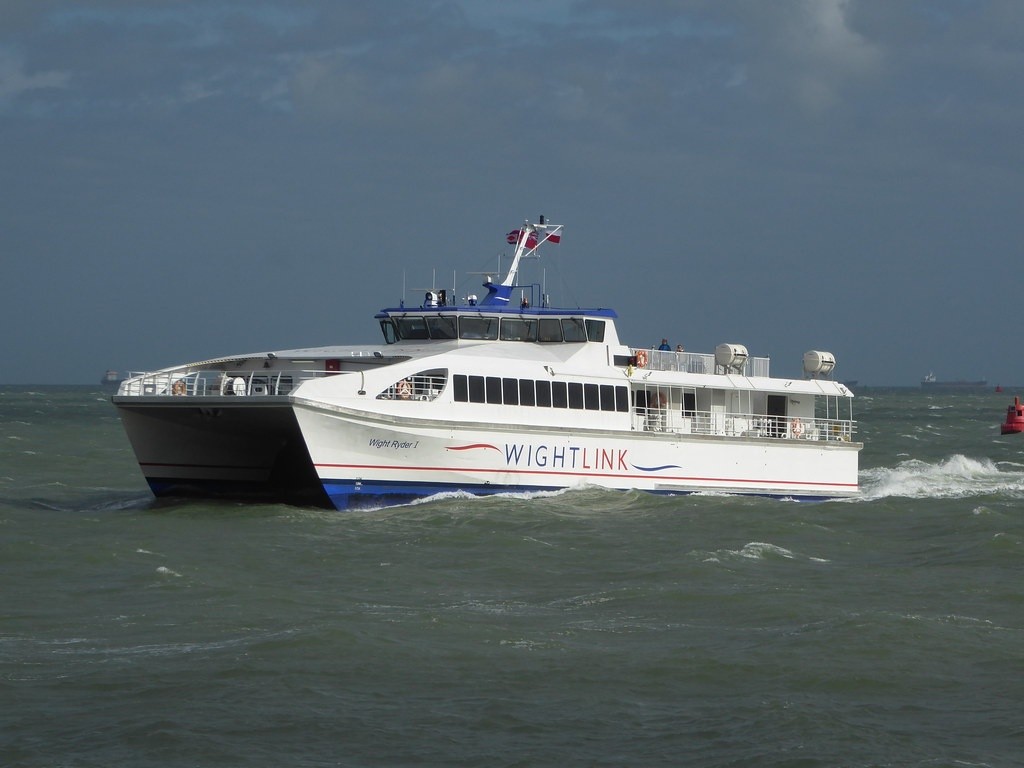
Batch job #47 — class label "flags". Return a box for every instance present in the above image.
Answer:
[505,228,539,251]
[545,229,562,244]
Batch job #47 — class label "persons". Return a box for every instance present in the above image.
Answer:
[677,344,685,352]
[657,338,671,351]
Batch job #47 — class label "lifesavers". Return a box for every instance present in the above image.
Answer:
[652,393,667,408]
[789,417,803,437]
[635,348,649,370]
[397,378,413,401]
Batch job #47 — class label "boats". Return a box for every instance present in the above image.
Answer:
[100,369,125,393]
[109,215,864,514]
[840,379,859,391]
[922,378,989,390]
